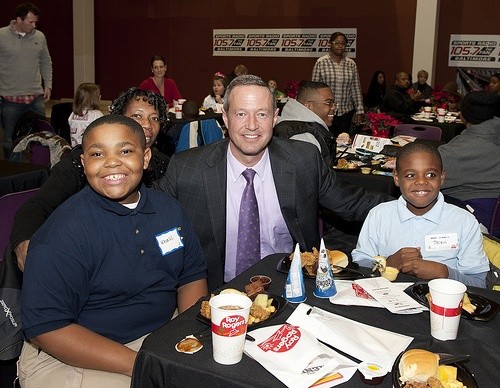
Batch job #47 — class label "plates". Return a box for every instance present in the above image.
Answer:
[410,283,500,322]
[436,116,457,121]
[194,291,288,331]
[411,112,435,122]
[276,255,359,279]
[332,151,395,172]
[391,349,479,388]
[447,112,459,116]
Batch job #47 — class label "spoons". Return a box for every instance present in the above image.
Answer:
[330,264,380,278]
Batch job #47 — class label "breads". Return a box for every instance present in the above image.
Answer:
[329,250,349,273]
[245,279,266,302]
[337,158,349,168]
[399,349,441,384]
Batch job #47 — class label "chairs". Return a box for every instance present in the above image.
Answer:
[0,98,500,336]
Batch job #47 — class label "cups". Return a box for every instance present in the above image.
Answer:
[428,278,467,341]
[361,167,371,174]
[424,106,445,123]
[249,275,272,291]
[357,362,388,385]
[208,294,252,365]
[173,99,186,119]
[108,106,114,115]
[379,266,399,281]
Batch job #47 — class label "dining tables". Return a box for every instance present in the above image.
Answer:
[332,140,399,176]
[170,103,225,124]
[412,108,464,141]
[130,252,500,388]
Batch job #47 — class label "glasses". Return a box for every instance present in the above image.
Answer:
[331,41,347,46]
[309,101,335,107]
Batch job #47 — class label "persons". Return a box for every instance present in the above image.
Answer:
[0,5,53,157]
[272,81,339,170]
[139,54,181,110]
[150,74,396,292]
[311,32,365,138]
[16,115,210,388]
[68,83,105,150]
[351,141,490,289]
[434,90,500,201]
[368,65,500,125]
[167,101,202,139]
[202,64,285,119]
[2,84,172,292]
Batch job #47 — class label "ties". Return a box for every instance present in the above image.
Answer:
[235,169,260,279]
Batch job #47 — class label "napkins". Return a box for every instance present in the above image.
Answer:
[287,302,414,371]
[329,279,430,310]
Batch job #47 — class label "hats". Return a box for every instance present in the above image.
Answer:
[461,91,494,124]
